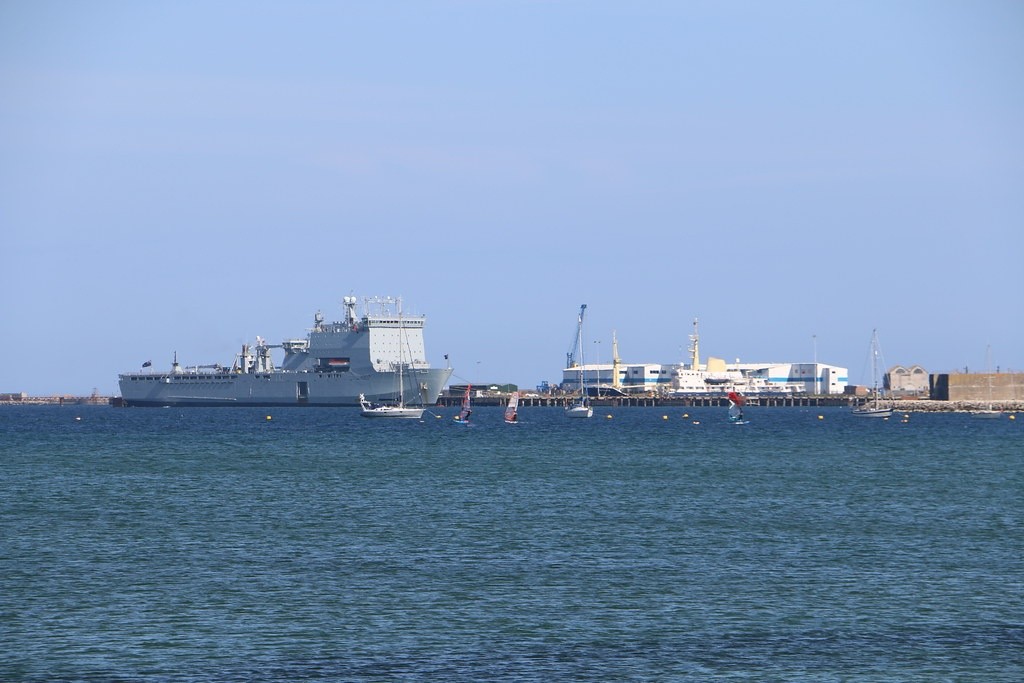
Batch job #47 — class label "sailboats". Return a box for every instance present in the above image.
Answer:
[504,391,519,422]
[358,310,427,419]
[969,345,1005,419]
[564,311,595,418]
[726,386,751,425]
[452,384,473,425]
[849,327,899,418]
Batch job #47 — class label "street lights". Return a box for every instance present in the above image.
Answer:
[593,339,602,396]
[811,334,820,396]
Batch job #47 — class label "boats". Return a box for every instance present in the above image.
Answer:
[662,317,807,397]
[116,286,455,409]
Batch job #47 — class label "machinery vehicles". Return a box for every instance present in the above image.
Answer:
[534,304,588,396]
